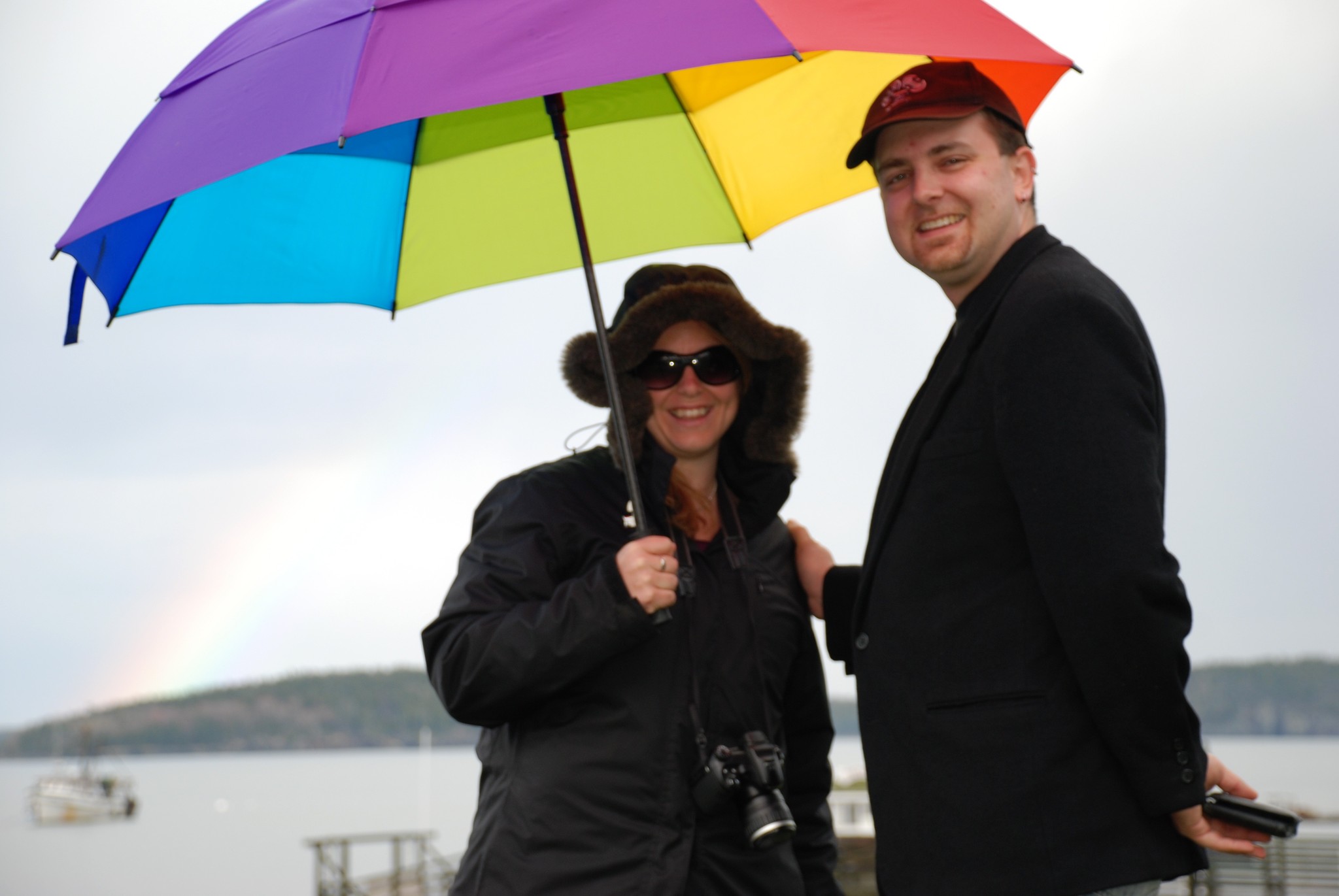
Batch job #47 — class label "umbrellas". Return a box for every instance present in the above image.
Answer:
[52,0,1086,625]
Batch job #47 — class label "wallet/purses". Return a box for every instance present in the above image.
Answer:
[1203,789,1299,838]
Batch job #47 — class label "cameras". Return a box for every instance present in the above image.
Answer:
[690,731,796,851]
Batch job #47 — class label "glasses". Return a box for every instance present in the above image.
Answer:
[630,345,742,391]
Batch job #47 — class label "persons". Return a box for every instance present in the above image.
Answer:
[781,59,1272,896]
[423,264,841,896]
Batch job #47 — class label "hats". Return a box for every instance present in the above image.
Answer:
[564,263,809,462]
[847,62,1026,170]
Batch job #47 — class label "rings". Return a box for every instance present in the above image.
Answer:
[660,556,665,571]
